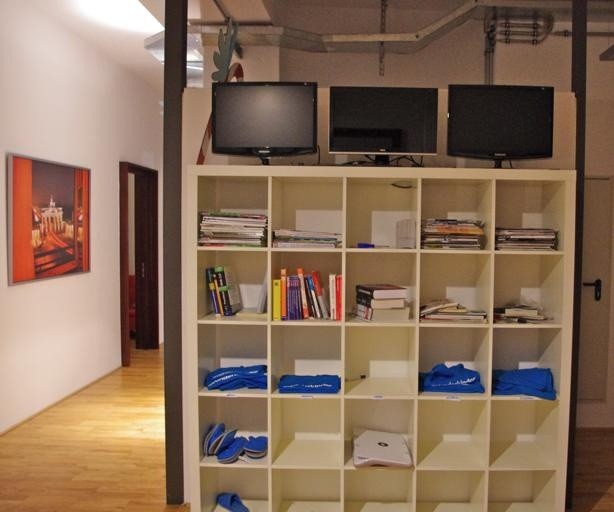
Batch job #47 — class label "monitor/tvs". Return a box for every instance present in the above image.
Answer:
[328,86,438,166]
[447,84,554,168]
[212,82,317,165]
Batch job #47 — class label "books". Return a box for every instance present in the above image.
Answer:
[494,304,555,324]
[422,217,486,251]
[206,267,224,317]
[419,298,488,324]
[272,227,341,249]
[198,208,268,248]
[215,266,243,316]
[352,303,410,322]
[352,427,413,468]
[356,284,408,298]
[495,227,559,252]
[272,267,342,321]
[356,296,404,310]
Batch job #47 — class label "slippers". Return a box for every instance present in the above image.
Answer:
[217,492,249,512]
[203,423,267,464]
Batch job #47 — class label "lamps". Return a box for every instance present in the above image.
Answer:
[144,31,205,73]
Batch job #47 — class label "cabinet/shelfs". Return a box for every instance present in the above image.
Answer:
[180,165,576,512]
